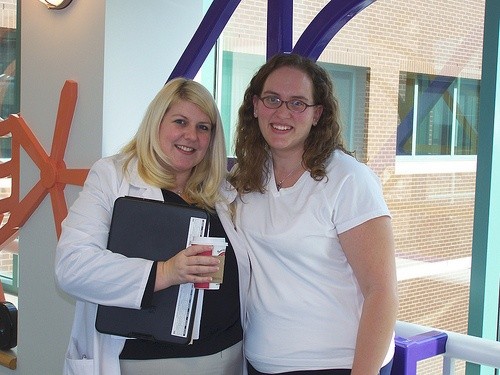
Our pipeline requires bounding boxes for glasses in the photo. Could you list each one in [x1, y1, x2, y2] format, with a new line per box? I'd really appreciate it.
[256, 95, 318, 113]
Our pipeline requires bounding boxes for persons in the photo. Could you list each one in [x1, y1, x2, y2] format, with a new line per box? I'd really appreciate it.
[211, 51, 399, 375]
[52, 77, 249, 375]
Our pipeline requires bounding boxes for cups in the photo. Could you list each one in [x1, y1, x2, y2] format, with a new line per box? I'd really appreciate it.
[190, 236, 228, 291]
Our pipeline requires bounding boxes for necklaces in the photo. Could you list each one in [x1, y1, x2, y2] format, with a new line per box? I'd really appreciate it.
[273, 159, 305, 190]
